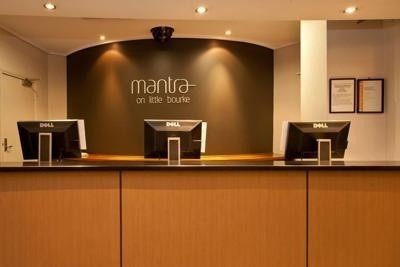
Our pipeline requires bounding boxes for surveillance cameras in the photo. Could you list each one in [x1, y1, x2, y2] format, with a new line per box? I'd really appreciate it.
[150, 26, 174, 43]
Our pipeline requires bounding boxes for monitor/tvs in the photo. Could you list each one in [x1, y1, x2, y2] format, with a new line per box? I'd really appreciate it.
[284, 121, 351, 165]
[144, 120, 203, 165]
[17, 121, 82, 167]
